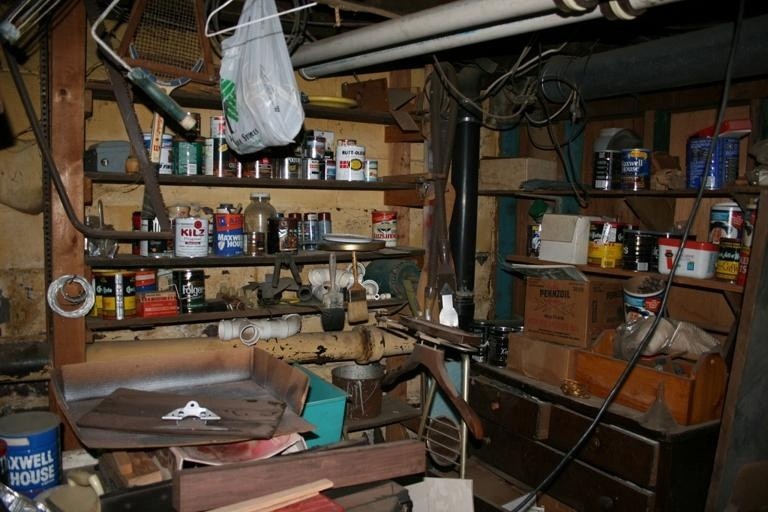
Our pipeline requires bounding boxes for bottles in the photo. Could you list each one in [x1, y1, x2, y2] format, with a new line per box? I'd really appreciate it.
[438, 294, 458, 327]
[131, 212, 141, 255]
[244, 193, 277, 253]
[288, 212, 303, 248]
[304, 213, 319, 250]
[318, 212, 332, 241]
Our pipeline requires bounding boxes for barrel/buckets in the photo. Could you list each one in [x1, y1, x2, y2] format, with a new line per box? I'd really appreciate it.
[622, 272, 669, 326]
[685, 137, 739, 189]
[332, 365, 385, 420]
[0, 411, 62, 499]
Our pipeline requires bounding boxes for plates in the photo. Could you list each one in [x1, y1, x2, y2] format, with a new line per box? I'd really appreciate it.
[307, 96, 358, 109]
[321, 234, 372, 243]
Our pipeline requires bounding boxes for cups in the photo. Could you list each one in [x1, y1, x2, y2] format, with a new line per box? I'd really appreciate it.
[167, 207, 192, 219]
[623, 275, 669, 321]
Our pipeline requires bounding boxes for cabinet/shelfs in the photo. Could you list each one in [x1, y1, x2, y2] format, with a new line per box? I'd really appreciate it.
[466, 352, 722, 511]
[508, 75, 768, 358]
[84, 72, 425, 334]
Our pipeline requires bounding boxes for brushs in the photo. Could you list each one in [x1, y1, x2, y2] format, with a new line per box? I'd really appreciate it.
[322, 252, 344, 332]
[348, 251, 368, 323]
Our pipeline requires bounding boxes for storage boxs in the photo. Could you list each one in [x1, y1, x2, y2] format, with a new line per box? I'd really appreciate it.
[300, 363, 352, 451]
[520, 267, 632, 351]
[506, 330, 578, 389]
[574, 326, 726, 425]
[537, 212, 619, 264]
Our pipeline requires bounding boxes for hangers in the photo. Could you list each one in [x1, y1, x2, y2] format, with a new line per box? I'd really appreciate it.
[203, 0, 319, 38]
[381, 342, 487, 442]
